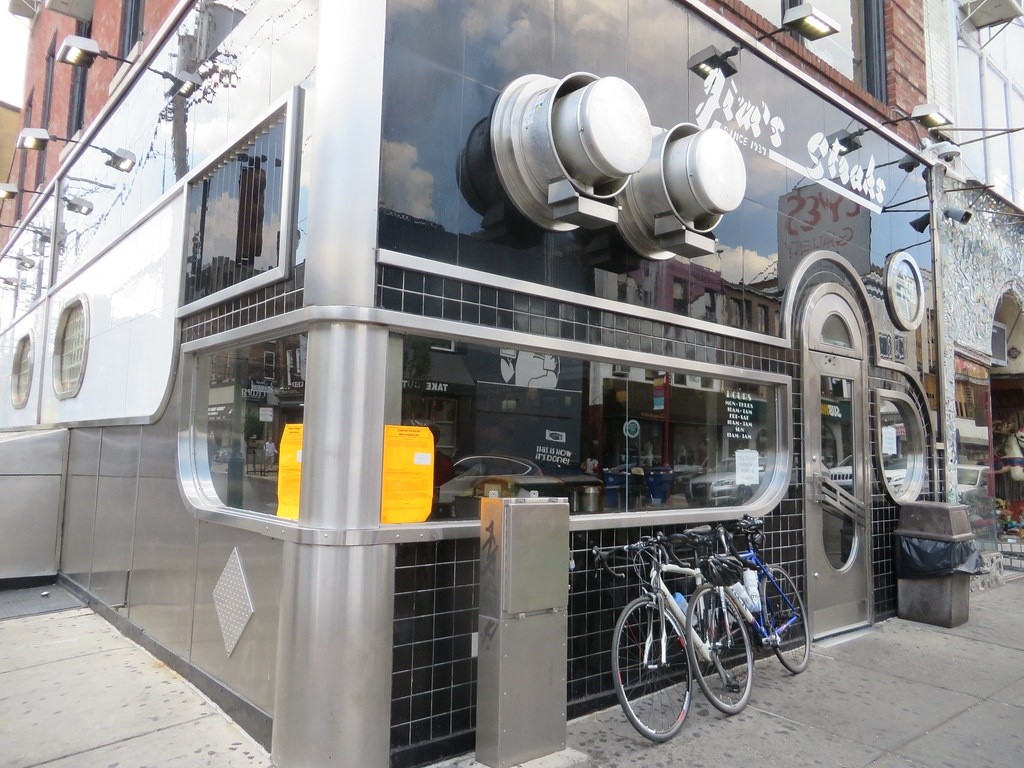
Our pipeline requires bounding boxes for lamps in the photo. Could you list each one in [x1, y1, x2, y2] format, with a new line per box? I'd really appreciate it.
[688, 45, 747, 80]
[25, 228, 67, 243]
[881, 103, 956, 128]
[15, 127, 78, 151]
[51, 193, 95, 215]
[826, 127, 871, 157]
[89, 143, 137, 173]
[0, 276, 19, 287]
[757, 3, 841, 42]
[4, 254, 35, 269]
[0, 182, 42, 199]
[54, 34, 134, 70]
[149, 68, 203, 99]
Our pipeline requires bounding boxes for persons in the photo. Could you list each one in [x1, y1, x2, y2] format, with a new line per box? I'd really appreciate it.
[263, 437, 278, 472]
[405, 419, 454, 519]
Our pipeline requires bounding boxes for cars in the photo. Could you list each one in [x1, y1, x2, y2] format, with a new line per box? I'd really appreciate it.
[432, 453, 604, 520]
[684, 456, 769, 507]
[609, 463, 703, 492]
[956, 464, 990, 503]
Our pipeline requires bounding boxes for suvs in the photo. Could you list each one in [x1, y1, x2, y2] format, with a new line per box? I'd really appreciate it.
[827, 454, 908, 495]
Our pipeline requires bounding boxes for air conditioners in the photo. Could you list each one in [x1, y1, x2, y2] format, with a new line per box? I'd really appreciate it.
[613, 364, 631, 374]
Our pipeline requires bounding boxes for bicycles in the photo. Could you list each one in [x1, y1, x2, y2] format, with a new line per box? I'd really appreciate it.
[591, 514, 811, 744]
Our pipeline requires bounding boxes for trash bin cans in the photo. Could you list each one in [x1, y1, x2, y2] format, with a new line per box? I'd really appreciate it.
[893, 500, 975, 628]
[604, 463, 673, 508]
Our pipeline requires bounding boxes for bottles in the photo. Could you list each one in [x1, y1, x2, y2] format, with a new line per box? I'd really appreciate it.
[568, 487, 601, 512]
[673, 592, 697, 627]
[728, 582, 755, 612]
[743, 568, 761, 612]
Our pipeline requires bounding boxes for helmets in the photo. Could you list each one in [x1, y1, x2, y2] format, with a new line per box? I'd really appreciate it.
[699, 556, 743, 586]
